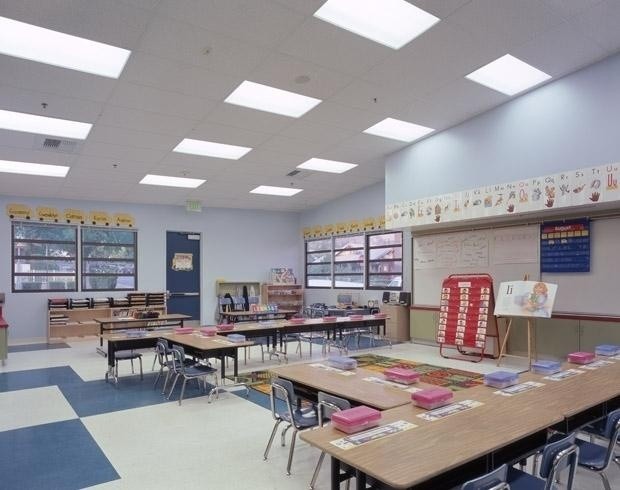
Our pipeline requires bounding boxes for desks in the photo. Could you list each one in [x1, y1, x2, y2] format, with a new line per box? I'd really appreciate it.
[298, 350, 619, 490]
[270, 353, 488, 412]
[0, 313, 10, 366]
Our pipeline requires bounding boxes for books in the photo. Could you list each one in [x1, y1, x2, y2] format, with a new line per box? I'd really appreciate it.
[46, 292, 167, 327]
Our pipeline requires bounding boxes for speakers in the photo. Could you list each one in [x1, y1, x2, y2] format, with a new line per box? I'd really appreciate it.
[382, 292, 411, 306]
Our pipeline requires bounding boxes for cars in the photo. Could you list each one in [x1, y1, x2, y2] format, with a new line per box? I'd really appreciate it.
[387, 276, 401, 288]
[13, 234, 135, 276]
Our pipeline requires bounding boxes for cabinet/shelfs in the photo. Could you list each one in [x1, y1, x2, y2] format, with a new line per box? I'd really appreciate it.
[47, 292, 167, 345]
[262, 283, 303, 314]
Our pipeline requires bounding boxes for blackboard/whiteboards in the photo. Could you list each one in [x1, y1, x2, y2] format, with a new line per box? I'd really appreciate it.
[410, 213, 620, 322]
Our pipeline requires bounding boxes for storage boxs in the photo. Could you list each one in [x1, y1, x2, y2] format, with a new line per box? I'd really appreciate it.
[567, 353, 596, 365]
[594, 344, 620, 356]
[327, 355, 358, 370]
[384, 367, 420, 385]
[330, 405, 382, 434]
[530, 360, 563, 376]
[411, 387, 453, 410]
[483, 370, 519, 389]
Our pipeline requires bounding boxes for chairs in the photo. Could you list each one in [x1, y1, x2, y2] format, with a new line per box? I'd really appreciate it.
[546, 410, 619, 490]
[92, 296, 390, 389]
[309, 391, 352, 490]
[165, 344, 218, 406]
[452, 463, 510, 490]
[506, 432, 580, 490]
[262, 377, 318, 476]
